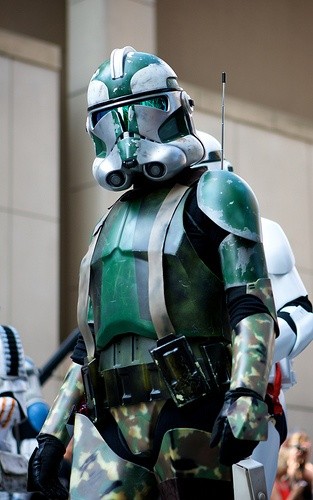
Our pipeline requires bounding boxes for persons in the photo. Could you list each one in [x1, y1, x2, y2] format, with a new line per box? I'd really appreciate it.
[192, 127, 312, 498]
[270, 430, 311, 500]
[0, 323, 73, 500]
[25, 46, 282, 500]
